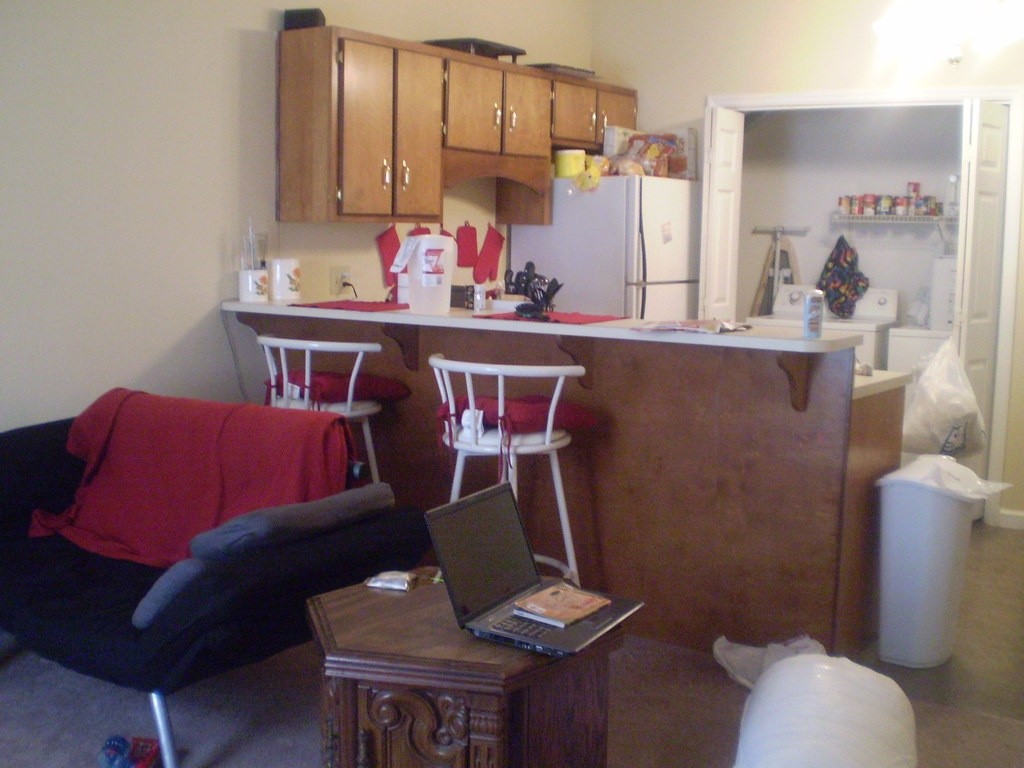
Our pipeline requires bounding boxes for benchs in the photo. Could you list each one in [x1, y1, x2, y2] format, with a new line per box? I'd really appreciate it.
[0, 387, 429, 768]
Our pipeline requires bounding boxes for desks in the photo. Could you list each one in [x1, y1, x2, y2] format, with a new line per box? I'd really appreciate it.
[308, 566, 626, 768]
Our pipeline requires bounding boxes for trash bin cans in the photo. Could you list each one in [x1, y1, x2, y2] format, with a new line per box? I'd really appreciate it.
[873, 454, 1015, 670]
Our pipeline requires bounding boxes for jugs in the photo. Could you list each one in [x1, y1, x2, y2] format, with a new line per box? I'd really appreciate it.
[406, 234, 459, 316]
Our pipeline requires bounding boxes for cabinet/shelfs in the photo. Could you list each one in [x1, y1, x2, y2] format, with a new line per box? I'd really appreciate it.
[277, 25, 638, 229]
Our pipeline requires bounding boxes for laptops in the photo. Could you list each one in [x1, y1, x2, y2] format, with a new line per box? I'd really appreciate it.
[425, 482, 647, 655]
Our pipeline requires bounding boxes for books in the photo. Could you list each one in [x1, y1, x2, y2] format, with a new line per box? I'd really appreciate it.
[514, 581, 610, 629]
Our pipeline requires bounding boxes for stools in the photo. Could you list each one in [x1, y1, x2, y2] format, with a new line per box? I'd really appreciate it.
[252, 334, 409, 483]
[430, 352, 602, 587]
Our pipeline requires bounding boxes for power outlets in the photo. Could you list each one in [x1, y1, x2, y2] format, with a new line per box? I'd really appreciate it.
[332, 255, 354, 295]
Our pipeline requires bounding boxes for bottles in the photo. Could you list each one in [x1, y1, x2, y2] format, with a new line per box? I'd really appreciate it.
[837, 183, 937, 218]
[464, 286, 489, 312]
[803, 288, 826, 336]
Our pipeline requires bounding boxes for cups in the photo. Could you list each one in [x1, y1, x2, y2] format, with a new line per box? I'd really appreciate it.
[553, 148, 585, 178]
[266, 258, 301, 306]
[397, 272, 410, 304]
[237, 269, 269, 307]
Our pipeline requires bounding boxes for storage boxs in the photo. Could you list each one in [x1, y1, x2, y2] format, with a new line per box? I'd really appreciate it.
[601, 124, 642, 154]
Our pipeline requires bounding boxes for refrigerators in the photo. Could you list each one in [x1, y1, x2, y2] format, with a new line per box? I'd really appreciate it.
[508, 177, 701, 322]
